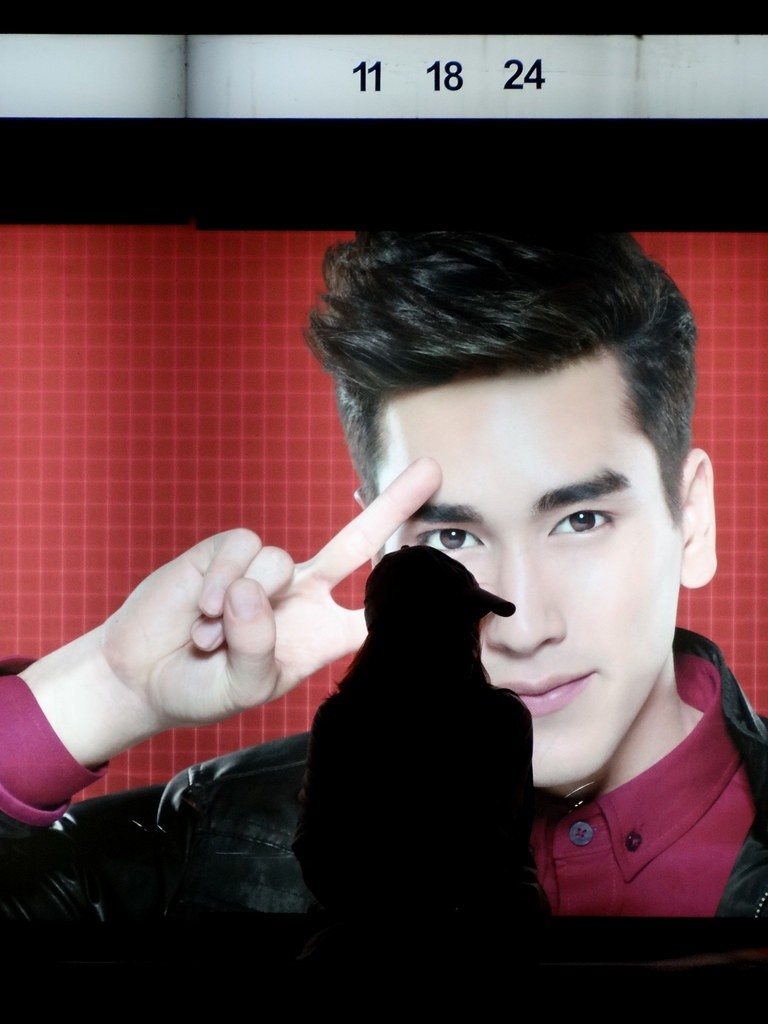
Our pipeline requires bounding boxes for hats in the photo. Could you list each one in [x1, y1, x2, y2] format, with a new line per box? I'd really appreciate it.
[364, 544, 516, 631]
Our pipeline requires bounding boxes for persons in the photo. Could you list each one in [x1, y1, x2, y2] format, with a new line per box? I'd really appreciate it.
[0, 229, 768, 924]
[295, 544, 553, 1024]
